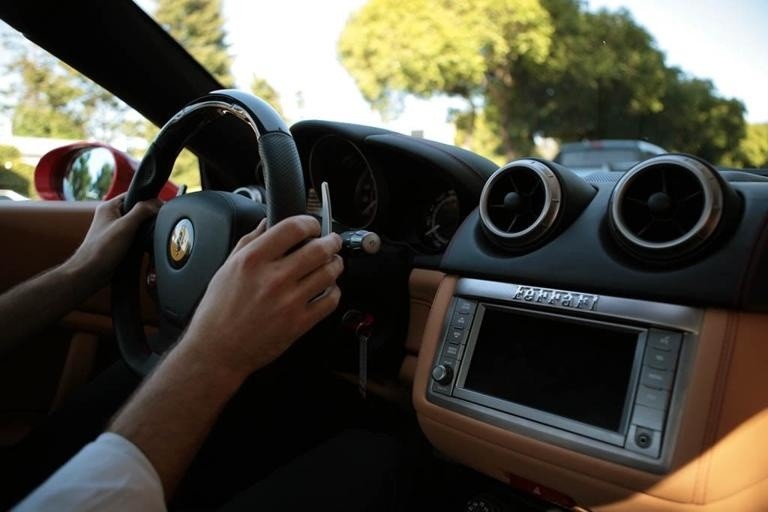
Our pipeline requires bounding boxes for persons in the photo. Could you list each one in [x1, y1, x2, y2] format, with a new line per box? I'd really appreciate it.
[2, 189, 346, 512]
